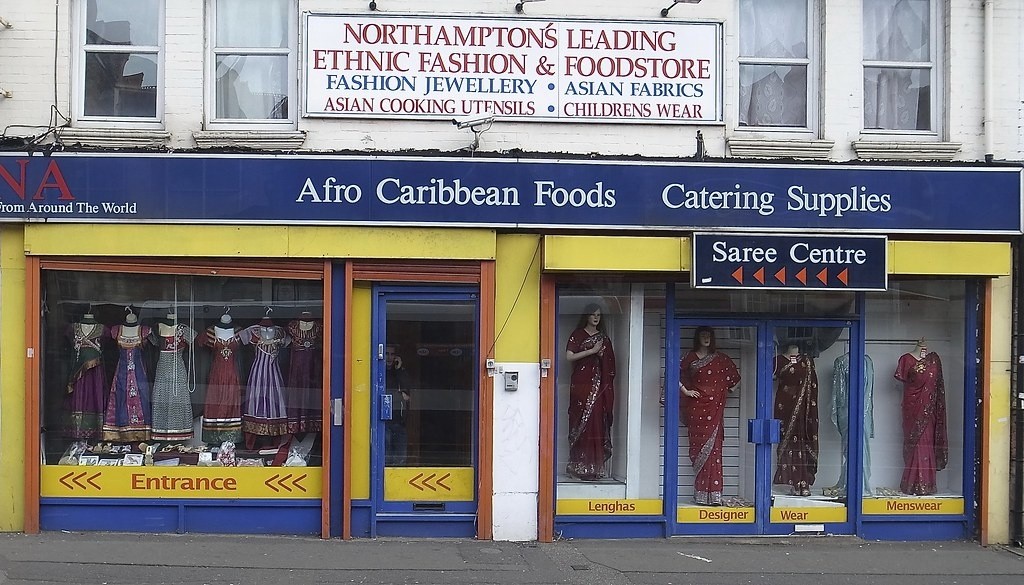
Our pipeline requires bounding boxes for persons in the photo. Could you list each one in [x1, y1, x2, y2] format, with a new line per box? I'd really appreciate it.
[199, 313, 245, 446]
[678, 325, 741, 507]
[773, 343, 821, 497]
[893, 341, 948, 495]
[386, 343, 411, 467]
[239, 314, 293, 438]
[286, 311, 323, 434]
[823, 340, 877, 496]
[66, 313, 105, 440]
[150, 313, 200, 441]
[565, 303, 617, 481]
[103, 313, 152, 444]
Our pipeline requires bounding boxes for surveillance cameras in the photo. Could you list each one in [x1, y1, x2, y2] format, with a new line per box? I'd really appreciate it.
[451, 112, 492, 129]
